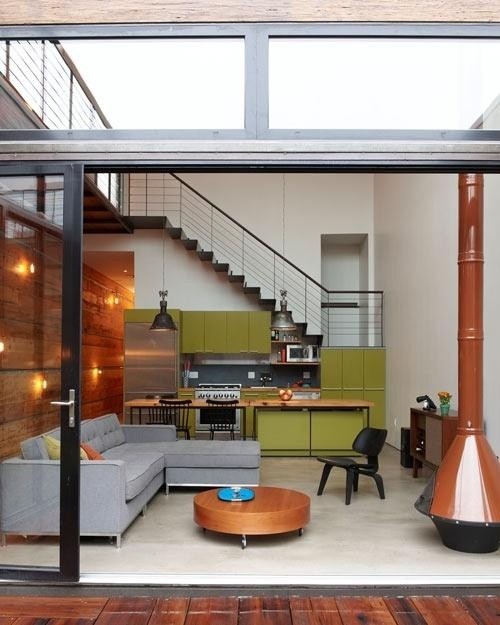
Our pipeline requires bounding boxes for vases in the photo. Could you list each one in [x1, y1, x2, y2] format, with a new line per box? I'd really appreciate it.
[439, 404, 450, 416]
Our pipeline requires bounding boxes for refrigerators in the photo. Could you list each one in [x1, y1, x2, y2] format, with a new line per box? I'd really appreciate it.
[123, 321, 180, 432]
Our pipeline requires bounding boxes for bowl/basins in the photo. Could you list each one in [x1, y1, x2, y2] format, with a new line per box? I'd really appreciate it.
[278, 393, 293, 401]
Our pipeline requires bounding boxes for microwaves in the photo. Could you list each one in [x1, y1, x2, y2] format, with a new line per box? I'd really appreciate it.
[287, 345, 321, 364]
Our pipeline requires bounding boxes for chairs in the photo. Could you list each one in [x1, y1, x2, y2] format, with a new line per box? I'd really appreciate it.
[206, 398, 239, 440]
[316, 427, 388, 504]
[145, 393, 192, 440]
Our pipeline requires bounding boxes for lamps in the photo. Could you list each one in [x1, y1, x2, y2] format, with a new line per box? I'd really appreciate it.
[269, 171, 298, 332]
[148, 174, 178, 331]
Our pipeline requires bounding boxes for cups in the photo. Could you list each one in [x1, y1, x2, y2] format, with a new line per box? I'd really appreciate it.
[233, 488, 241, 493]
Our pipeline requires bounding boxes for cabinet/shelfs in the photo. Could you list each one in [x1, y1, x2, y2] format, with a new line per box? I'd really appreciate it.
[256, 410, 311, 457]
[227, 311, 271, 354]
[271, 340, 320, 366]
[319, 347, 387, 430]
[241, 392, 280, 438]
[311, 410, 363, 456]
[180, 311, 227, 354]
[409, 406, 458, 478]
[179, 392, 195, 437]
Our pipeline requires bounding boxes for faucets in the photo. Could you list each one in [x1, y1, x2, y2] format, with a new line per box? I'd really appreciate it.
[259, 374, 273, 387]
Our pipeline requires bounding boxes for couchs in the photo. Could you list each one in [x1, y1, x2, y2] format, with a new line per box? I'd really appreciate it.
[1, 424, 261, 549]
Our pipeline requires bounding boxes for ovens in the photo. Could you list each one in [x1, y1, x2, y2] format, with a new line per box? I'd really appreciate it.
[196, 384, 241, 431]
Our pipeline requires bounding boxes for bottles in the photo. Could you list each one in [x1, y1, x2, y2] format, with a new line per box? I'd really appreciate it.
[271, 330, 281, 341]
[281, 350, 284, 362]
[283, 333, 298, 341]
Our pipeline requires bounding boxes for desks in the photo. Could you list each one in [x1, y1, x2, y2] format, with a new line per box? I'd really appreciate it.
[124, 398, 250, 441]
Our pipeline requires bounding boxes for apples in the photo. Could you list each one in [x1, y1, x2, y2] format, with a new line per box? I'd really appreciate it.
[279, 389, 293, 400]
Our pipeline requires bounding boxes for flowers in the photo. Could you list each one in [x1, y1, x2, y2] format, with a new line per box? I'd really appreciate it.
[438, 392, 452, 405]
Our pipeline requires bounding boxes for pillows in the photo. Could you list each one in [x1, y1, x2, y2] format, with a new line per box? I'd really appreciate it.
[39, 433, 105, 461]
